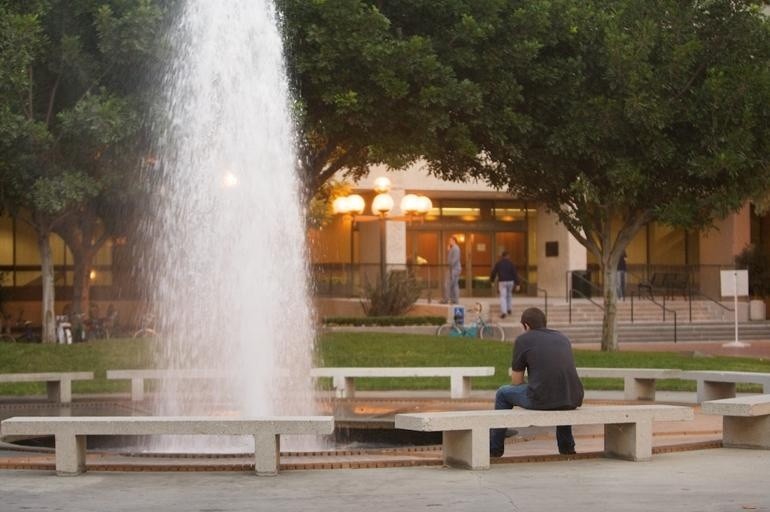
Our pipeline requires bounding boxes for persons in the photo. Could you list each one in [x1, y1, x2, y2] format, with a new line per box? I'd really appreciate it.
[616, 251, 628, 302]
[439, 237, 462, 304]
[491, 251, 515, 317]
[489, 306, 585, 457]
[63, 304, 120, 341]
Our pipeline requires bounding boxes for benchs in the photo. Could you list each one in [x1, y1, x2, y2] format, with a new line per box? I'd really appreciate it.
[0, 371, 94, 403]
[105, 370, 276, 402]
[509, 367, 681, 402]
[393, 404, 694, 470]
[701, 394, 770, 451]
[1, 415, 335, 478]
[682, 369, 770, 404]
[309, 366, 495, 399]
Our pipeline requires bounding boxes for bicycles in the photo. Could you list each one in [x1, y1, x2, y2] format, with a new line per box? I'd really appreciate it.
[1, 304, 163, 346]
[433, 302, 508, 343]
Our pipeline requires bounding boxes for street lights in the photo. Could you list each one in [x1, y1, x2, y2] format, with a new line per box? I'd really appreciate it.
[332, 177, 435, 294]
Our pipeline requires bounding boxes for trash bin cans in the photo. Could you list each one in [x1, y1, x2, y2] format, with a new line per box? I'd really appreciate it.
[572, 270, 592, 298]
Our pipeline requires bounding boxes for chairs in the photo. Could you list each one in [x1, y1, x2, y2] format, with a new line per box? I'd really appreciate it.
[638, 271, 690, 302]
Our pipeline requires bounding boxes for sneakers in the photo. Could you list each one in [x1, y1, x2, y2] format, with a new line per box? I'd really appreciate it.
[490, 451, 504, 457]
[560, 449, 577, 454]
[437, 298, 460, 304]
[500, 309, 512, 320]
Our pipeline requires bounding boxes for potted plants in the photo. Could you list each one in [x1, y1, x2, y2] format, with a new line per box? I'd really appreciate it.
[734, 241, 770, 321]
